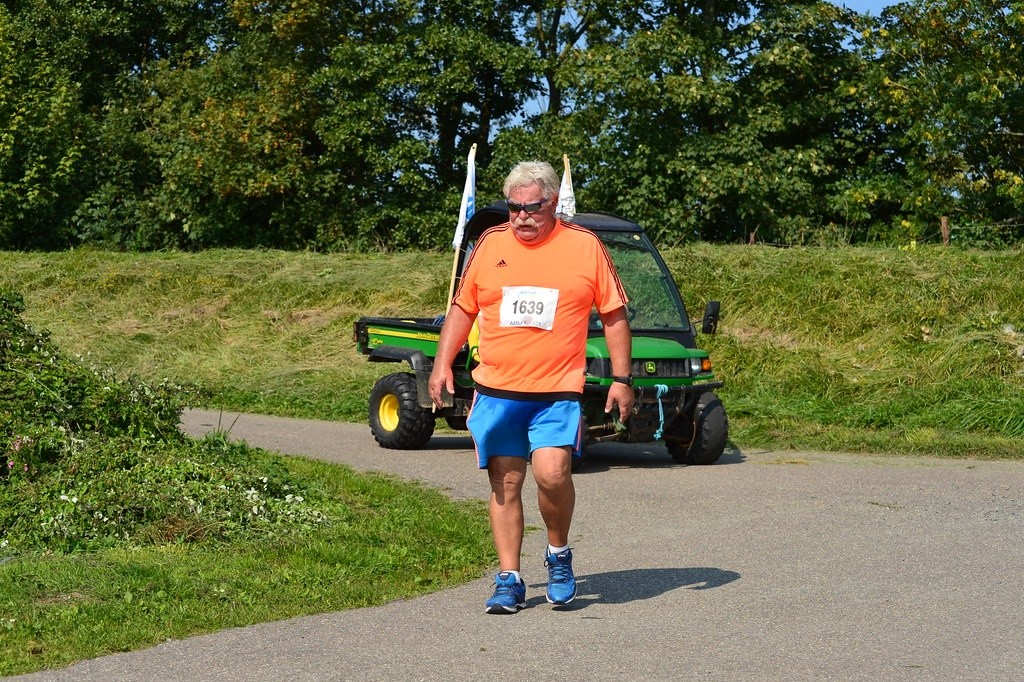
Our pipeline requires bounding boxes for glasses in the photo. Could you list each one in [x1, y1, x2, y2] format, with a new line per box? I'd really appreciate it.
[505, 197, 549, 213]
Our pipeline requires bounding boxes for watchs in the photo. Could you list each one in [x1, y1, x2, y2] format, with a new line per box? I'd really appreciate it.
[612, 374, 634, 387]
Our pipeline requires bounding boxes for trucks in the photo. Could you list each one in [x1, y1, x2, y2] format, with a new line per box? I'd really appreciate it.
[353, 202, 729, 463]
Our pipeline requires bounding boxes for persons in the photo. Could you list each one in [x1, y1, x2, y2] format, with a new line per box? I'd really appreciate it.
[428, 159, 635, 614]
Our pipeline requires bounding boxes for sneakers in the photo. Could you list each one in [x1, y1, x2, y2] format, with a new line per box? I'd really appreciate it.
[544, 545, 577, 606]
[485, 572, 527, 614]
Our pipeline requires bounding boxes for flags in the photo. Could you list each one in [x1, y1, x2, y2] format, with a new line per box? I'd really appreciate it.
[452, 143, 477, 251]
[555, 154, 576, 217]
